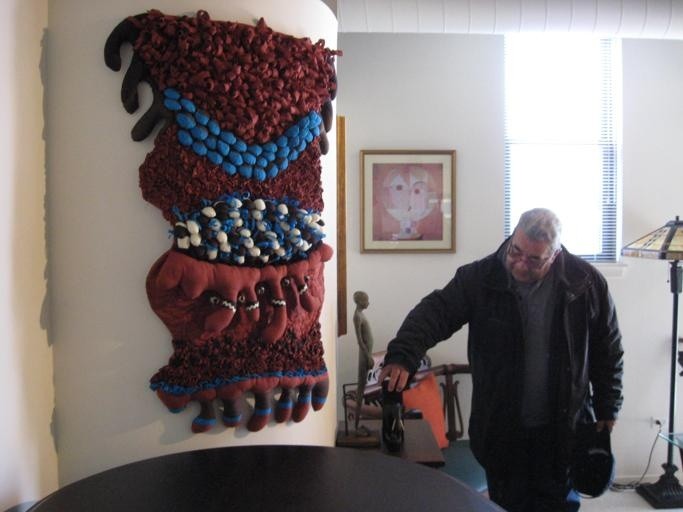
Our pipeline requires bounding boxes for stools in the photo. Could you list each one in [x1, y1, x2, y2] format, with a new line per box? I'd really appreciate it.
[434, 432, 493, 495]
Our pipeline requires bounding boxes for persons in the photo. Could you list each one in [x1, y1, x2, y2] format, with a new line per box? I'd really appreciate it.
[378, 208, 624, 512]
[352, 291, 374, 437]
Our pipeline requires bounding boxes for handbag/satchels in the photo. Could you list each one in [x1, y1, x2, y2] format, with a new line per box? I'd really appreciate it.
[568, 391, 615, 497]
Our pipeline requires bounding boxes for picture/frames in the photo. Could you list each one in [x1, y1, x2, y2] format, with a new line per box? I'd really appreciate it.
[357, 147, 458, 258]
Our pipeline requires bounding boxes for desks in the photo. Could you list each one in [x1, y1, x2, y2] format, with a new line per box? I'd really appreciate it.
[4, 434, 517, 511]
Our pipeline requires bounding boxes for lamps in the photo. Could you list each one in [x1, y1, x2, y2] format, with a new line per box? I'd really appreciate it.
[616, 203, 683, 510]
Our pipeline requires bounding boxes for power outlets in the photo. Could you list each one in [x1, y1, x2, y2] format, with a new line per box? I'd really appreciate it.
[650, 417, 666, 430]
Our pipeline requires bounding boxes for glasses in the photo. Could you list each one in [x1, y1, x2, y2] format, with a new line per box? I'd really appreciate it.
[508, 241, 552, 267]
